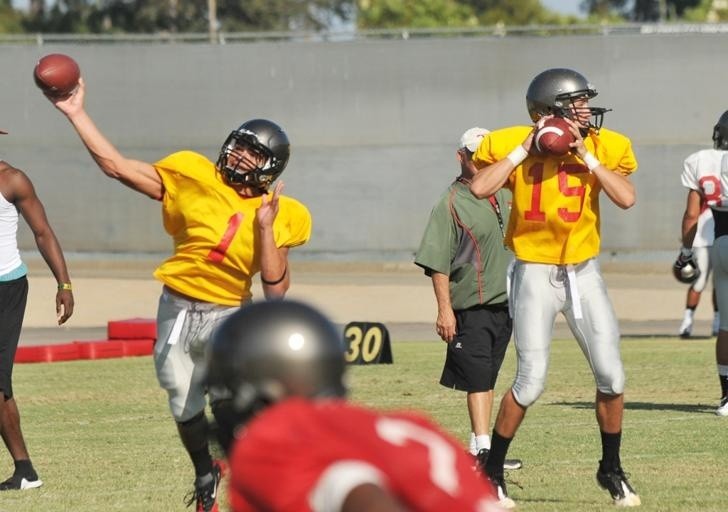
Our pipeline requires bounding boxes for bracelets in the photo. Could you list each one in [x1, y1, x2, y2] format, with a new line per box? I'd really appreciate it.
[56, 283, 72, 291]
[258, 266, 288, 286]
[582, 151, 601, 170]
[507, 144, 528, 168]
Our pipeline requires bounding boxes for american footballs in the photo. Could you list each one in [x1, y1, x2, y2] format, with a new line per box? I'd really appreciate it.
[535, 117, 575, 156]
[34, 54, 80, 95]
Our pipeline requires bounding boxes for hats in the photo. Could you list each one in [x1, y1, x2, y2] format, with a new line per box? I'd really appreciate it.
[459, 127, 491, 152]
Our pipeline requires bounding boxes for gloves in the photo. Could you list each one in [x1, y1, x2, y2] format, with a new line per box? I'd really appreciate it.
[672, 255, 701, 283]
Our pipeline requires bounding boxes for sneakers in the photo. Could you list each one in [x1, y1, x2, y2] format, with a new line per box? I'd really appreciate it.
[467, 449, 522, 470]
[679, 311, 720, 339]
[493, 479, 515, 508]
[184, 460, 226, 512]
[717, 401, 728, 416]
[0, 471, 43, 490]
[597, 460, 641, 507]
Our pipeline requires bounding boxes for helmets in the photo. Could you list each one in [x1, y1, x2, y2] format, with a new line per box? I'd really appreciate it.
[712, 110, 728, 151]
[215, 118, 291, 195]
[197, 299, 346, 452]
[525, 68, 612, 135]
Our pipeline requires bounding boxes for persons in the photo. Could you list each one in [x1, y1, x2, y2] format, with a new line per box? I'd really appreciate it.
[206, 298, 516, 512]
[0, 130, 74, 492]
[469, 69, 642, 509]
[43, 76, 312, 512]
[413, 127, 523, 471]
[671, 110, 728, 417]
[678, 200, 720, 337]
